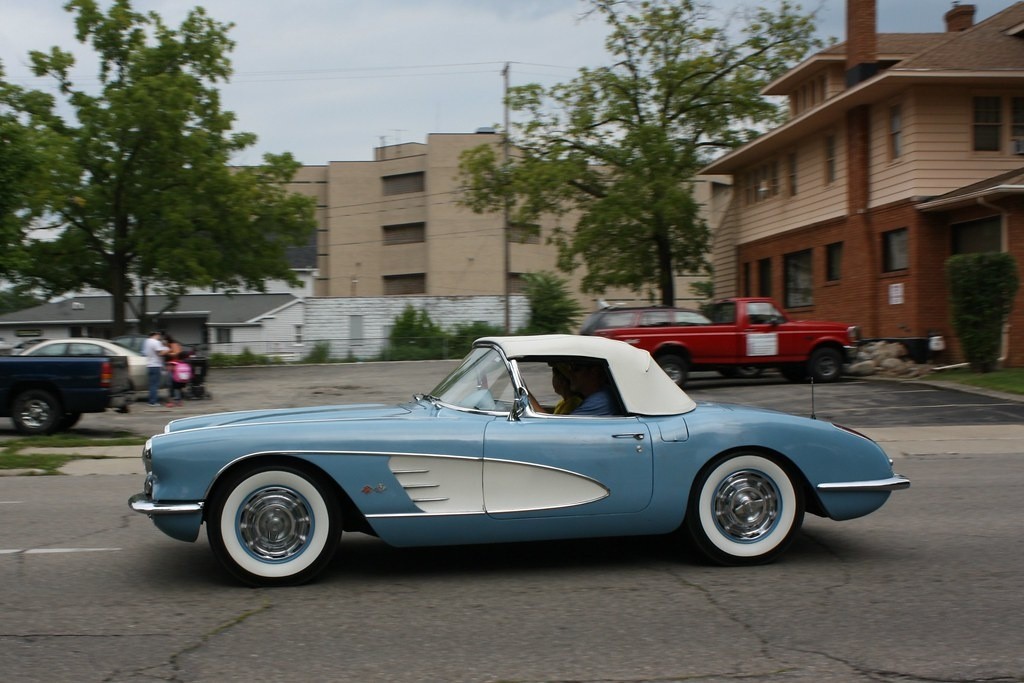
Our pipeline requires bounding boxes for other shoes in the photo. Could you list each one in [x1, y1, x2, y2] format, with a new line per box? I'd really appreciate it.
[148, 403, 161, 406]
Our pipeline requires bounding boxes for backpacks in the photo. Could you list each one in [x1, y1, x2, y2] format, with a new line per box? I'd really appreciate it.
[171, 362, 192, 383]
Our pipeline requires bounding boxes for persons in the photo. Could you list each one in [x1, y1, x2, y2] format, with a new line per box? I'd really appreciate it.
[142, 331, 168, 406]
[529, 357, 613, 415]
[161, 335, 186, 400]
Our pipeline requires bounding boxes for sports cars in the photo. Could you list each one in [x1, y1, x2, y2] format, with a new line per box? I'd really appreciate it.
[128, 334, 912, 588]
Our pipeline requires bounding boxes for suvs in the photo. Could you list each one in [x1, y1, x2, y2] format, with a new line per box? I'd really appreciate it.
[579, 306, 765, 380]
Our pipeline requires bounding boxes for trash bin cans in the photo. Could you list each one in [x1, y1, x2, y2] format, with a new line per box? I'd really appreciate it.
[183, 356, 208, 399]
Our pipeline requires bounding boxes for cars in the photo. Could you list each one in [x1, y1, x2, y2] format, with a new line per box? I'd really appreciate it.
[2, 337, 167, 406]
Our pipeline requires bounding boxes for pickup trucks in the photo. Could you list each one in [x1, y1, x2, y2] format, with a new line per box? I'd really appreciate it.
[591, 298, 862, 392]
[0, 353, 130, 435]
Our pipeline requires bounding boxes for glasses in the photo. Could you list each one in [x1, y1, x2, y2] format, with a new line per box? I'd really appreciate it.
[569, 364, 599, 373]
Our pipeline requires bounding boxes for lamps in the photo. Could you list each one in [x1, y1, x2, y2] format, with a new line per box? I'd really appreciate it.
[756, 179, 778, 192]
